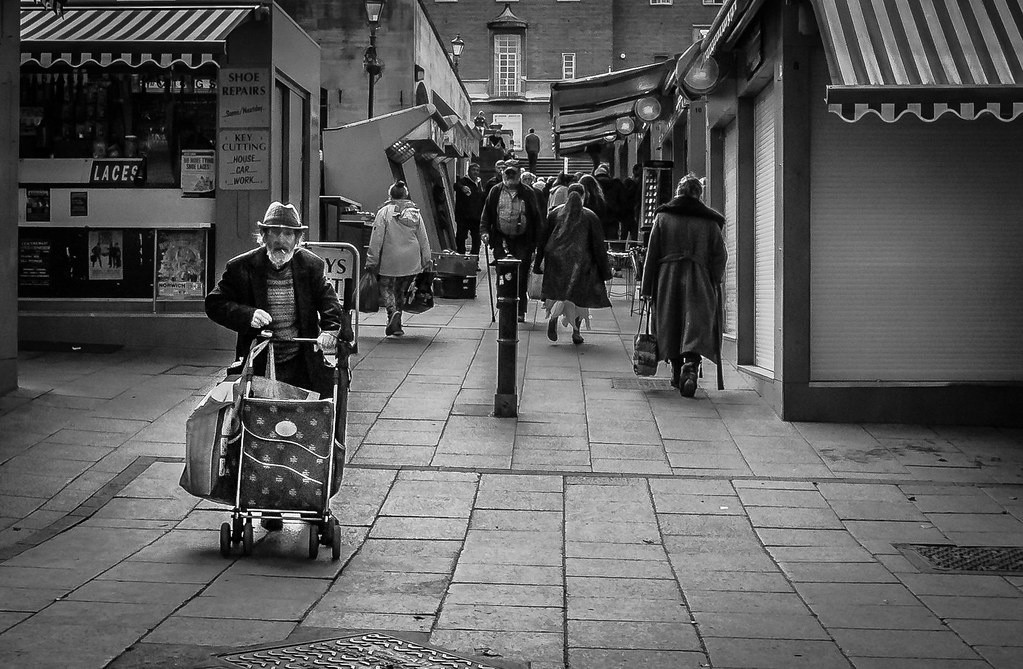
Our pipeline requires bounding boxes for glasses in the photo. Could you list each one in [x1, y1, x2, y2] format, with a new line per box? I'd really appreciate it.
[523, 178, 530, 180]
[506, 172, 517, 175]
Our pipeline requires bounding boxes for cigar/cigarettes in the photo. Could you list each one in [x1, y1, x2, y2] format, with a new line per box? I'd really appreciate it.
[280, 248, 286, 255]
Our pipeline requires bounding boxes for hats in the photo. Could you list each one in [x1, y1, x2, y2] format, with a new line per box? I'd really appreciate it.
[504, 159, 520, 173]
[256, 201, 309, 230]
[495, 160, 506, 167]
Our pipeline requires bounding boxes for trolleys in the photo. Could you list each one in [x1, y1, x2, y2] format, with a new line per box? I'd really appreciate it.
[220, 330, 341, 561]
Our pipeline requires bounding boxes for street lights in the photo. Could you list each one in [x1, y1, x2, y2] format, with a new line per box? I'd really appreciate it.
[449, 32, 466, 68]
[363, 0, 386, 116]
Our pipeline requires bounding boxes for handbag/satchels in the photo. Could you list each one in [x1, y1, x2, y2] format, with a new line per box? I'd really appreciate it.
[186, 346, 242, 495]
[351, 269, 379, 312]
[252, 341, 321, 400]
[404, 266, 436, 314]
[633, 299, 658, 376]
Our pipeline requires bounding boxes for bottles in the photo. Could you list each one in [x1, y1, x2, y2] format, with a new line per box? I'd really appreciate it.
[124, 135, 137, 158]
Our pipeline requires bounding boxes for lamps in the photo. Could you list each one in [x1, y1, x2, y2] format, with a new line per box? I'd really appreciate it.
[616, 116, 636, 136]
[633, 96, 663, 124]
[681, 55, 722, 96]
[451, 32, 466, 72]
[414, 65, 424, 82]
[603, 130, 618, 142]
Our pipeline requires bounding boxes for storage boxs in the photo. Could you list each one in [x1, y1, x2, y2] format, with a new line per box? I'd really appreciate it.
[431, 252, 479, 299]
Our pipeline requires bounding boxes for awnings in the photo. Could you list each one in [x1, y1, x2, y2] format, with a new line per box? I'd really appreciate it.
[20, 5, 266, 68]
[814, 0, 1023, 122]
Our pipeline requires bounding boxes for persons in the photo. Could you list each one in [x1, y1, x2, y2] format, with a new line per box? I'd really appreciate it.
[525, 129, 541, 174]
[203, 202, 343, 531]
[456, 164, 482, 272]
[480, 159, 622, 274]
[365, 181, 432, 336]
[487, 129, 505, 150]
[620, 164, 643, 250]
[474, 112, 488, 136]
[537, 184, 613, 343]
[479, 159, 541, 322]
[639, 171, 725, 397]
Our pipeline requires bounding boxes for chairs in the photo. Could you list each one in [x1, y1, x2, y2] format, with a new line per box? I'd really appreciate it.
[630, 248, 648, 315]
[606, 251, 631, 300]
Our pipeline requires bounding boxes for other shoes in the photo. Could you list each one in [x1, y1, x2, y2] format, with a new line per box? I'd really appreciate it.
[385, 309, 403, 336]
[548, 318, 558, 341]
[572, 333, 584, 343]
[669, 362, 685, 387]
[518, 311, 524, 322]
[614, 270, 622, 277]
[533, 266, 543, 274]
[679, 362, 698, 397]
[261, 513, 283, 531]
[301, 508, 339, 526]
